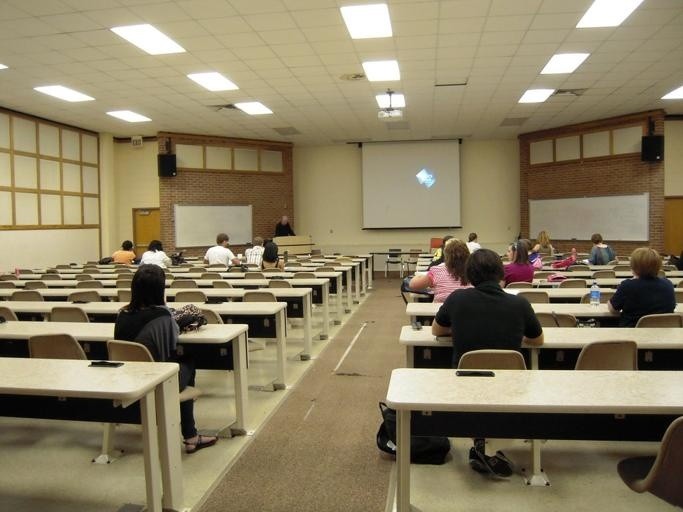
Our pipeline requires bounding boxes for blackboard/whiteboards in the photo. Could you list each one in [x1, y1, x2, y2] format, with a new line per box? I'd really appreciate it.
[529, 195, 651, 242]
[174, 204, 252, 248]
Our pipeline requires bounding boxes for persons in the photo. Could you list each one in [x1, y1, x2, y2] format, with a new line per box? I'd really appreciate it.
[589, 234, 615, 265]
[524, 238, 542, 262]
[274, 215, 296, 237]
[435, 245, 443, 256]
[139, 240, 172, 268]
[203, 233, 240, 267]
[427, 236, 454, 271]
[112, 240, 137, 265]
[531, 231, 553, 256]
[465, 232, 481, 254]
[431, 249, 544, 478]
[113, 264, 219, 454]
[262, 242, 284, 272]
[499, 240, 534, 288]
[245, 237, 266, 269]
[607, 247, 676, 328]
[410, 241, 475, 304]
[438, 238, 461, 266]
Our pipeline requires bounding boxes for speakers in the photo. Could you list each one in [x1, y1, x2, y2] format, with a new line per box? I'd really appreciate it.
[642, 135, 664, 160]
[156, 153, 176, 179]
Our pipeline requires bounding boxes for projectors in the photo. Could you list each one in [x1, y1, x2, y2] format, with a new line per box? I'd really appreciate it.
[379, 91, 404, 121]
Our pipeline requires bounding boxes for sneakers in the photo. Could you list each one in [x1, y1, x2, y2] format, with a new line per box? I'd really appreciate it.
[469, 448, 490, 474]
[474, 441, 513, 478]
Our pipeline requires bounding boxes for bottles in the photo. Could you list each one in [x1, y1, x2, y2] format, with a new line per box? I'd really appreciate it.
[571, 248, 577, 262]
[590, 282, 600, 312]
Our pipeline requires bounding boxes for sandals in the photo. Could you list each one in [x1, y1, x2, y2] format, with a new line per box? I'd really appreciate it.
[183, 435, 218, 454]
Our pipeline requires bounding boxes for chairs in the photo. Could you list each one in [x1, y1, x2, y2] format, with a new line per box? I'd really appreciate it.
[569, 265, 591, 272]
[673, 292, 683, 304]
[311, 255, 324, 258]
[0, 282, 15, 289]
[40, 274, 61, 280]
[15, 269, 33, 274]
[199, 308, 225, 325]
[201, 272, 222, 280]
[75, 275, 94, 281]
[117, 274, 134, 280]
[534, 312, 578, 328]
[262, 267, 282, 272]
[227, 266, 245, 273]
[27, 334, 88, 361]
[241, 264, 259, 268]
[24, 282, 48, 290]
[209, 264, 226, 268]
[612, 265, 631, 272]
[0, 307, 18, 322]
[457, 349, 527, 371]
[188, 267, 207, 273]
[45, 269, 59, 274]
[385, 248, 402, 279]
[10, 291, 44, 302]
[212, 281, 232, 289]
[82, 268, 100, 274]
[293, 272, 316, 279]
[505, 282, 533, 289]
[82, 265, 97, 269]
[515, 291, 549, 304]
[163, 273, 175, 280]
[316, 267, 335, 272]
[114, 270, 132, 274]
[242, 292, 278, 302]
[55, 264, 72, 269]
[404, 249, 423, 276]
[324, 262, 341, 266]
[335, 256, 352, 263]
[101, 339, 202, 464]
[268, 281, 292, 288]
[635, 313, 683, 329]
[0, 275, 17, 281]
[574, 341, 638, 371]
[114, 264, 131, 269]
[660, 264, 678, 272]
[76, 281, 103, 288]
[676, 280, 683, 288]
[617, 416, 683, 509]
[287, 254, 297, 259]
[170, 280, 198, 288]
[244, 272, 265, 280]
[162, 267, 170, 272]
[580, 291, 616, 304]
[116, 281, 132, 289]
[533, 272, 557, 279]
[295, 259, 312, 263]
[87, 262, 97, 265]
[50, 307, 90, 323]
[117, 290, 132, 302]
[541, 263, 552, 266]
[180, 264, 194, 268]
[174, 291, 208, 303]
[285, 262, 301, 267]
[67, 291, 102, 303]
[591, 271, 615, 279]
[558, 280, 587, 289]
[540, 256, 557, 262]
[615, 256, 630, 262]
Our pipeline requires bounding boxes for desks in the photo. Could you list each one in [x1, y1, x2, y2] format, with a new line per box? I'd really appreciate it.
[183, 258, 367, 297]
[0, 301, 288, 392]
[0, 357, 183, 512]
[413, 254, 683, 286]
[0, 320, 249, 439]
[184, 262, 360, 304]
[385, 367, 683, 512]
[30, 268, 343, 325]
[406, 302, 683, 325]
[0, 274, 330, 341]
[0, 287, 314, 361]
[409, 288, 683, 302]
[67, 265, 353, 313]
[180, 254, 373, 290]
[399, 325, 683, 487]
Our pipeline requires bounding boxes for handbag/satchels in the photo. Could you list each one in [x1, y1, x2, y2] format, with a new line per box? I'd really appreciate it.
[400, 274, 434, 305]
[551, 255, 576, 268]
[99, 256, 112, 265]
[226, 264, 248, 272]
[169, 303, 207, 333]
[170, 249, 187, 266]
[547, 274, 567, 282]
